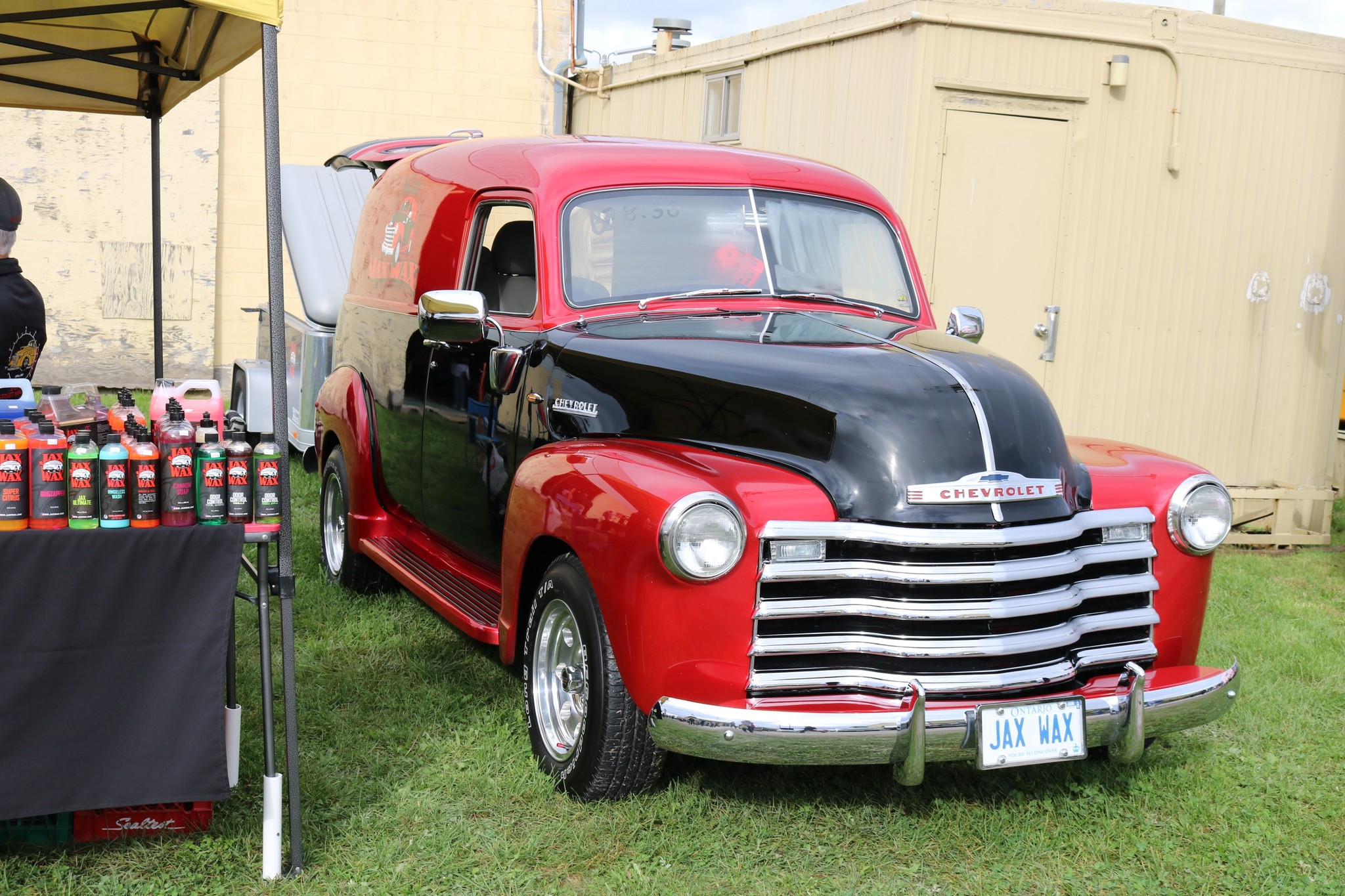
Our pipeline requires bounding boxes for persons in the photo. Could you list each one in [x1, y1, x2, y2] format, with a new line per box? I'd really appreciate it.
[0, 178, 46, 399]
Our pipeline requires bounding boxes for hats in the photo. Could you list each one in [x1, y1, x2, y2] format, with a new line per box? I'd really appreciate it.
[0, 177, 22, 231]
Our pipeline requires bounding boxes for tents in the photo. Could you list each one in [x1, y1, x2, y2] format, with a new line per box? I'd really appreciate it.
[0, 0, 303, 448]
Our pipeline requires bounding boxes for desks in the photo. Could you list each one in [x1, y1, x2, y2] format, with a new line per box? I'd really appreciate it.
[0, 515, 281, 883]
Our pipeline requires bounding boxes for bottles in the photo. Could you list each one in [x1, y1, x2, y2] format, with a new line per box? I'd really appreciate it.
[252, 433, 282, 524]
[13, 408, 38, 429]
[108, 387, 146, 434]
[196, 433, 226, 526]
[68, 435, 99, 529]
[203, 411, 211, 420]
[220, 430, 236, 449]
[0, 419, 28, 531]
[225, 432, 253, 524]
[21, 414, 45, 437]
[78, 429, 99, 473]
[76, 433, 99, 486]
[155, 397, 197, 526]
[120, 413, 160, 528]
[17, 411, 42, 430]
[28, 422, 69, 529]
[26, 419, 64, 435]
[195, 419, 219, 459]
[99, 434, 130, 528]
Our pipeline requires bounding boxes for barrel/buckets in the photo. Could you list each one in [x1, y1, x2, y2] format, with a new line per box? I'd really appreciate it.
[149, 378, 224, 446]
[0, 378, 36, 422]
[36, 382, 109, 453]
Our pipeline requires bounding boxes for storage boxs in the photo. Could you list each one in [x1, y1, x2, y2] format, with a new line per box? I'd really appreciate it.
[0, 802, 213, 854]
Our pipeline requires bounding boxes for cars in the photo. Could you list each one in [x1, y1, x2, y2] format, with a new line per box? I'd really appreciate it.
[301, 129, 1243, 804]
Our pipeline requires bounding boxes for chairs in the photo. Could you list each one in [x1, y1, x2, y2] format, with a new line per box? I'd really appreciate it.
[474, 221, 610, 313]
